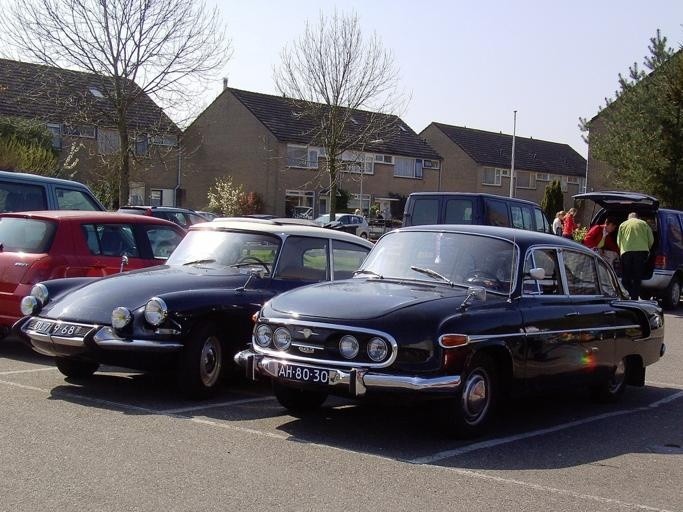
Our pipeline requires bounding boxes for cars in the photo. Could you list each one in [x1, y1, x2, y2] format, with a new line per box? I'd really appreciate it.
[0, 211, 190, 355]
[103, 205, 401, 246]
[232, 222, 666, 441]
[15, 215, 375, 398]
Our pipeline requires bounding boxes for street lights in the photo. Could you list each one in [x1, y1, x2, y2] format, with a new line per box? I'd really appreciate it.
[358, 140, 382, 215]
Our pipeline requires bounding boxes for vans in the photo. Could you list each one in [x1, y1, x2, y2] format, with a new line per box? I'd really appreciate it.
[568, 190, 682, 309]
[402, 192, 562, 281]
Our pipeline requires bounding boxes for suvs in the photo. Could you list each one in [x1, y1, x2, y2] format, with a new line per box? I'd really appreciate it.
[0, 170, 134, 259]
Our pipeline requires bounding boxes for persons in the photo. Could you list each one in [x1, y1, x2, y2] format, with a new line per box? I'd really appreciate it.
[615, 211, 652, 301]
[581, 216, 616, 252]
[562, 208, 580, 239]
[376, 210, 384, 223]
[351, 209, 365, 222]
[552, 210, 565, 238]
